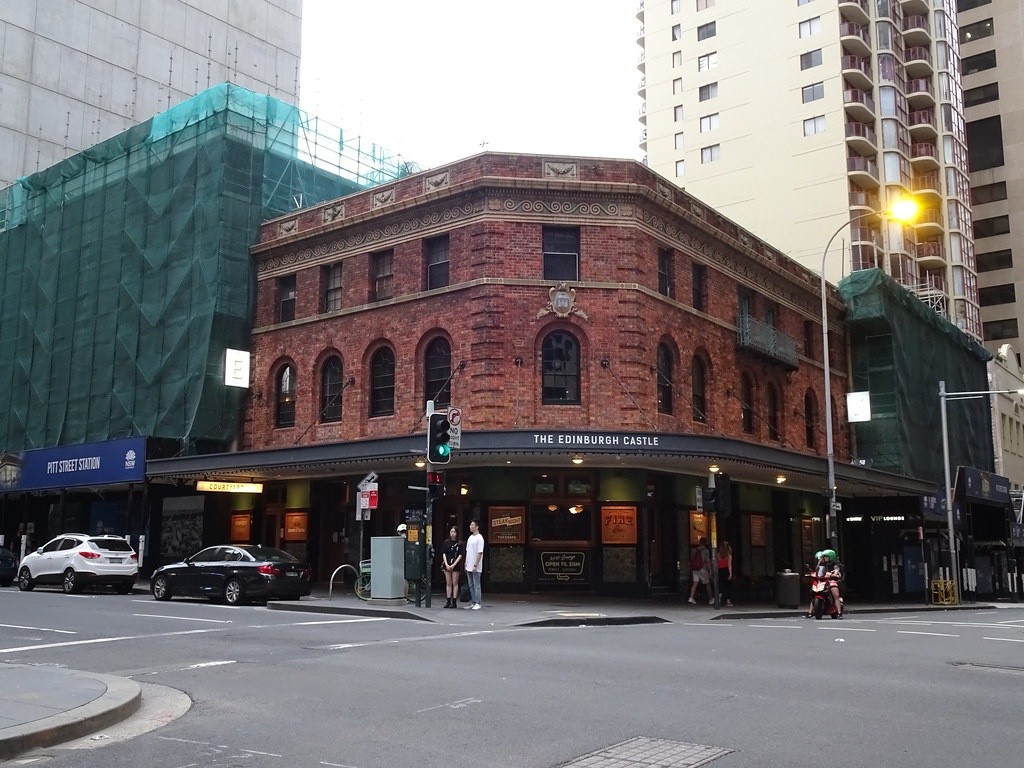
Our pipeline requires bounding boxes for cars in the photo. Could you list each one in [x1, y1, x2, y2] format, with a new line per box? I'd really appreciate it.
[151, 544, 315, 603]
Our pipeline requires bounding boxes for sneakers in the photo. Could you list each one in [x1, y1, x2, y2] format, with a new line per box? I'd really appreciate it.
[472, 603, 481, 610]
[463, 604, 474, 609]
[708, 598, 715, 604]
[689, 597, 698, 604]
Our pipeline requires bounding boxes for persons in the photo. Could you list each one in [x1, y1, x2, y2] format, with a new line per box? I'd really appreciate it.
[396, 523, 408, 538]
[806, 550, 844, 619]
[715, 539, 735, 606]
[801, 550, 842, 615]
[685, 537, 716, 605]
[439, 525, 465, 608]
[462, 519, 485, 610]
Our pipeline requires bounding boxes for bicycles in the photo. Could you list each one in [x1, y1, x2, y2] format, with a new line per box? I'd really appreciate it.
[355, 559, 427, 604]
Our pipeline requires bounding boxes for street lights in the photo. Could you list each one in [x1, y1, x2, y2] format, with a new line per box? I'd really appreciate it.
[822, 196, 924, 562]
[940, 381, 1024, 605]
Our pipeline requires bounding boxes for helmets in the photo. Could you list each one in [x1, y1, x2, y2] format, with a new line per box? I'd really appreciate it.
[815, 551, 824, 560]
[822, 549, 837, 562]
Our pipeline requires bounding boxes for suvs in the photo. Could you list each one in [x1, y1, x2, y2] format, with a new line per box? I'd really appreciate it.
[18, 532, 139, 594]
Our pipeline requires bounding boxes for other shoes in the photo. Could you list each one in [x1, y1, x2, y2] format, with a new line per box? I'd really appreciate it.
[443, 603, 451, 607]
[837, 614, 843, 619]
[449, 604, 456, 608]
[805, 614, 813, 618]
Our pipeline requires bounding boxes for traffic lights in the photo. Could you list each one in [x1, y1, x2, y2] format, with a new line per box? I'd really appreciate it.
[428, 413, 451, 464]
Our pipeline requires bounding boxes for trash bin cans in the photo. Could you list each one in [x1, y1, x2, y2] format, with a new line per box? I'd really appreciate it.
[777, 570, 802, 609]
